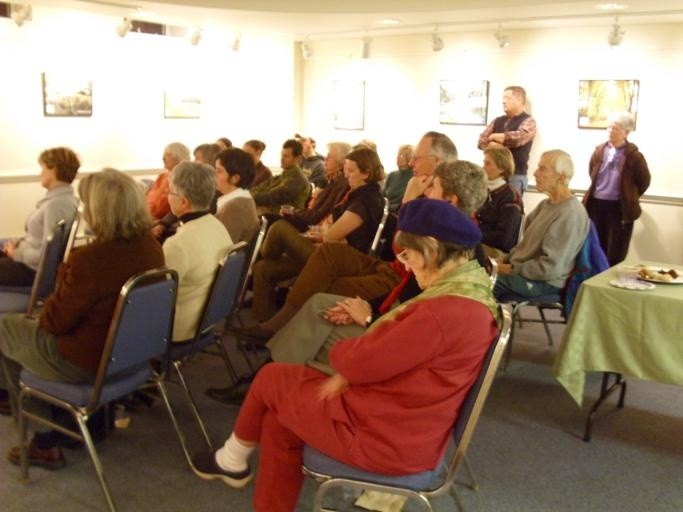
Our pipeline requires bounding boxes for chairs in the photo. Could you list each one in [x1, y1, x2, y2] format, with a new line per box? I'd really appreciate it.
[225, 218, 270, 371]
[302, 304, 512, 512]
[164, 241, 251, 449]
[0, 221, 65, 318]
[368, 197, 389, 256]
[510, 295, 563, 344]
[305, 183, 315, 208]
[14, 269, 193, 512]
[486, 257, 498, 290]
[0, 204, 83, 318]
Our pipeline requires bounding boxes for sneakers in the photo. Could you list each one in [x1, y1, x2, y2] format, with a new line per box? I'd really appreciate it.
[190, 452, 253, 489]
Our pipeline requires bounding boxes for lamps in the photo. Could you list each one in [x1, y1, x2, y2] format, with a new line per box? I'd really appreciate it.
[362, 38, 372, 58]
[495, 29, 510, 50]
[12, 4, 32, 27]
[232, 35, 241, 52]
[191, 28, 202, 44]
[431, 34, 444, 50]
[116, 19, 132, 37]
[609, 25, 625, 46]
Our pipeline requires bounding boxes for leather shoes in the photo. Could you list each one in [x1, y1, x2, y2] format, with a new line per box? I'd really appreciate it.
[0, 397, 11, 416]
[227, 323, 274, 342]
[205, 376, 251, 406]
[8, 439, 67, 470]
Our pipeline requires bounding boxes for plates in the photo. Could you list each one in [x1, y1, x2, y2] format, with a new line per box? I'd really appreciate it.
[635, 266, 682, 285]
[609, 278, 656, 292]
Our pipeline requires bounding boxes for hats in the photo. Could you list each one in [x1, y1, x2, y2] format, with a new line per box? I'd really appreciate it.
[398, 199, 483, 245]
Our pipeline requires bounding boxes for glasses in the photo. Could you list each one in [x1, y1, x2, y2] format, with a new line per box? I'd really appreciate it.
[412, 154, 435, 162]
[398, 250, 434, 265]
[163, 187, 180, 199]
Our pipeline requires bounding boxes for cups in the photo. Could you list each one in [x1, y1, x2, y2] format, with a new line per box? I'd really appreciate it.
[281, 205, 294, 215]
[616, 265, 639, 284]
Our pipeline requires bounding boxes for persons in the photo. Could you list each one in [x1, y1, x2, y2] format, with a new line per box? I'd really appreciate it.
[189, 195, 496, 512]
[492, 152, 591, 305]
[582, 109, 651, 270]
[7, 169, 165, 472]
[476, 86, 537, 196]
[0, 132, 521, 410]
